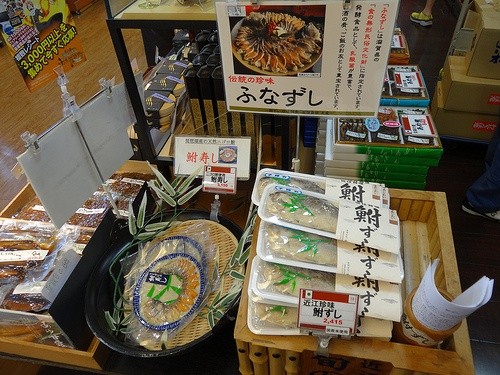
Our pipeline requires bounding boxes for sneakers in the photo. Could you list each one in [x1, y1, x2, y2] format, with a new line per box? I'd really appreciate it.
[410, 11, 433, 26]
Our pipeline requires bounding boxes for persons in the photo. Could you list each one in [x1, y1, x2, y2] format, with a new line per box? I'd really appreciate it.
[409, 0, 436, 27]
[462, 133, 500, 223]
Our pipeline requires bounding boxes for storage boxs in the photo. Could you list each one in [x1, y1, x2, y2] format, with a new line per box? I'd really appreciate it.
[183, 28, 444, 191]
[0, 161, 157, 370]
[431, 0, 500, 141]
[234, 188, 477, 375]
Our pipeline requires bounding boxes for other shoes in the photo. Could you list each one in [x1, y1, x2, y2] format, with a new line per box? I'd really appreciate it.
[462, 196, 500, 222]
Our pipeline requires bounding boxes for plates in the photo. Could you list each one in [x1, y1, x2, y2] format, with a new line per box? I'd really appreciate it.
[231, 18, 323, 76]
[247, 168, 403, 339]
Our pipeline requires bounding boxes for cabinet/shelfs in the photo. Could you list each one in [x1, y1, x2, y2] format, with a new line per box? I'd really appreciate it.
[104, 0, 290, 187]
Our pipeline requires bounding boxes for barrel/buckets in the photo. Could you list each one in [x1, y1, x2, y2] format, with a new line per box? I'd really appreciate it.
[83, 206, 249, 374]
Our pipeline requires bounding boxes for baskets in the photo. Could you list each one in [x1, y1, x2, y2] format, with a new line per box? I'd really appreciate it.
[122, 219, 245, 351]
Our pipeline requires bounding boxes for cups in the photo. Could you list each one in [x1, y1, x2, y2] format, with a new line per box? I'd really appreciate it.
[393, 286, 462, 348]
